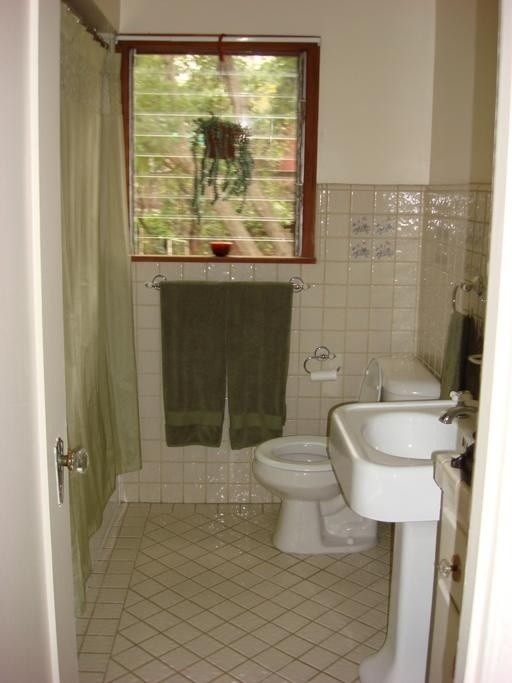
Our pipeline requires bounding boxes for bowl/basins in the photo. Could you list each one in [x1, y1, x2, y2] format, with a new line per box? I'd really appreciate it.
[210, 242, 233, 255]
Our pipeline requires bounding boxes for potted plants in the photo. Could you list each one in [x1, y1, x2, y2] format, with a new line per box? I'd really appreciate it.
[188, 110, 253, 230]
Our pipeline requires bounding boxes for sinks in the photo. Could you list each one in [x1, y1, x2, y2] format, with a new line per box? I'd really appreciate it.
[325, 399, 477, 522]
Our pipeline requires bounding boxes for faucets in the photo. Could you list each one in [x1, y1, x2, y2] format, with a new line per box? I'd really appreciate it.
[437, 404, 478, 424]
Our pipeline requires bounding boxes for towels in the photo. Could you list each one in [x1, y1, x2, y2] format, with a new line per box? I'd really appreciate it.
[157, 279, 287, 445]
[440, 308, 468, 404]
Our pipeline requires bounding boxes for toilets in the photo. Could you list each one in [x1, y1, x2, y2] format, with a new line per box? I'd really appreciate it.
[252, 356, 442, 556]
